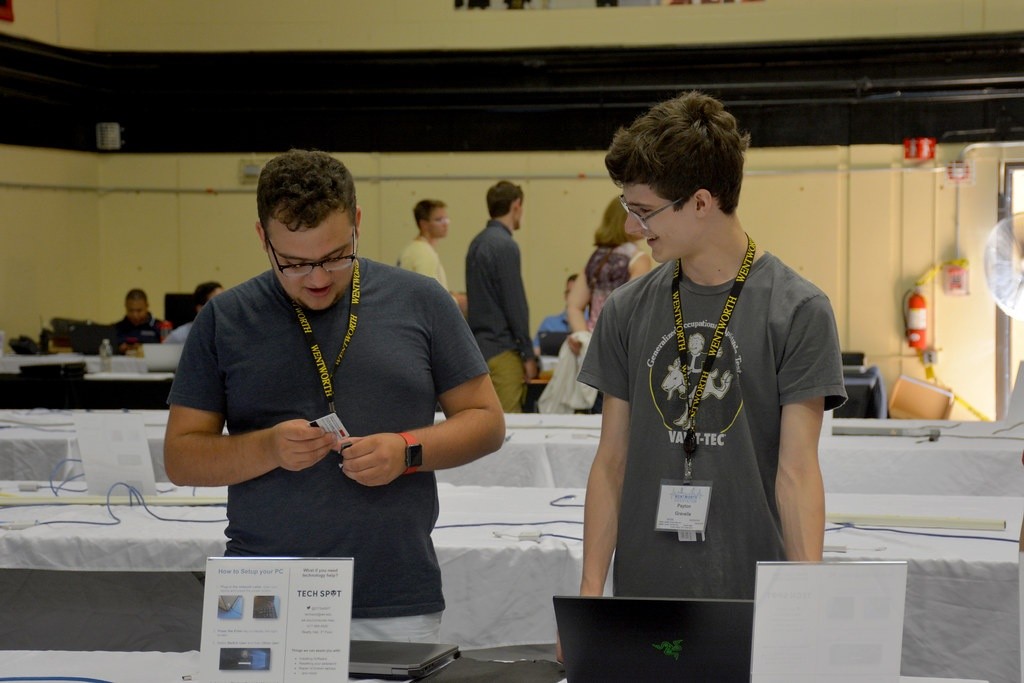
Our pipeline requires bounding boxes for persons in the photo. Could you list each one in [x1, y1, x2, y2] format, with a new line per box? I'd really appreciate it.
[109, 180, 652, 412]
[556, 91, 847, 664]
[163, 149, 506, 644]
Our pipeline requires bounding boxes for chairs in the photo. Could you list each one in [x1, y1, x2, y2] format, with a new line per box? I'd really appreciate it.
[82, 373, 174, 411]
[22, 363, 86, 410]
[886, 374, 956, 420]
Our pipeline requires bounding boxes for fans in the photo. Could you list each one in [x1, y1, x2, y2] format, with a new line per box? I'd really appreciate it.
[984, 213, 1024, 321]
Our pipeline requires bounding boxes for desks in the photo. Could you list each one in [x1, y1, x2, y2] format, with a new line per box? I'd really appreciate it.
[0, 410, 1024, 497]
[1, 650, 990, 683]
[0, 352, 147, 410]
[529, 367, 887, 420]
[0, 480, 1024, 683]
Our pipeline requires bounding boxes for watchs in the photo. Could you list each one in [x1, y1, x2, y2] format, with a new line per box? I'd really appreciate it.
[395, 432, 422, 475]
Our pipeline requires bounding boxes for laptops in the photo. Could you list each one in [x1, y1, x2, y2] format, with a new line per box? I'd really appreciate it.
[349, 639, 459, 679]
[554, 595, 755, 683]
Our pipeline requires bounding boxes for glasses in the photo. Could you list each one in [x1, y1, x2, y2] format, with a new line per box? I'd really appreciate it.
[618, 190, 697, 230]
[261, 217, 355, 278]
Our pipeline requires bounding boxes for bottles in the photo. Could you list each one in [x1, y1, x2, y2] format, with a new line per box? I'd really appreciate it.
[40, 316, 54, 355]
[99, 338, 112, 373]
